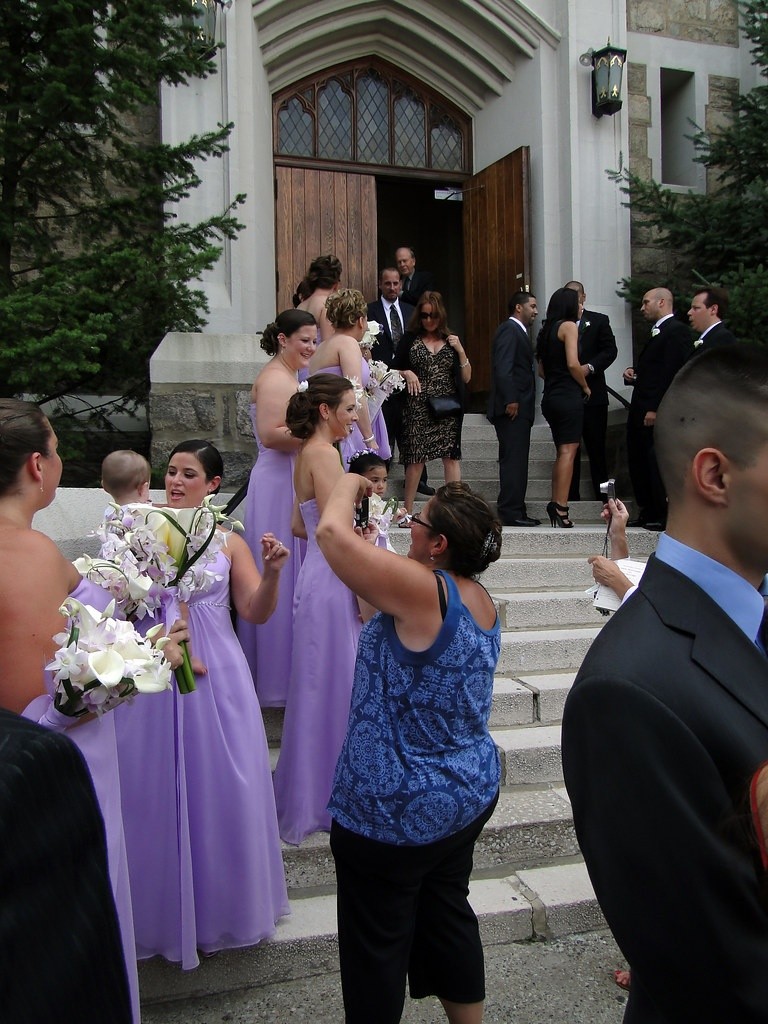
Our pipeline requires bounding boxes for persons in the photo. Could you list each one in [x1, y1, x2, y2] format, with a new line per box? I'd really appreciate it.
[316, 474, 503, 1024]
[561, 336, 768, 1024]
[366, 268, 437, 496]
[564, 281, 617, 500]
[588, 496, 639, 991]
[0, 252, 406, 1024]
[680, 287, 738, 369]
[485, 288, 544, 528]
[394, 248, 438, 306]
[536, 288, 591, 528]
[624, 287, 690, 532]
[391, 290, 472, 527]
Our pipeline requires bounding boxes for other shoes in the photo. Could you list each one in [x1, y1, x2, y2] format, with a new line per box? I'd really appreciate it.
[397, 514, 415, 528]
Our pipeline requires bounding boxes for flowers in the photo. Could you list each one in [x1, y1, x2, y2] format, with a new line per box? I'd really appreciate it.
[580, 320, 591, 336]
[44, 494, 245, 723]
[358, 320, 384, 350]
[368, 496, 399, 525]
[648, 328, 661, 339]
[693, 340, 704, 350]
[297, 379, 309, 392]
[344, 359, 406, 411]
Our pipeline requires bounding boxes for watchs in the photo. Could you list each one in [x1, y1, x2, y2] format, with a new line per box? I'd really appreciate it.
[588, 363, 595, 374]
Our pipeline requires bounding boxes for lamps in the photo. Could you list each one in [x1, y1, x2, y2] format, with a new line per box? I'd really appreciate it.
[181, 0, 233, 48]
[580, 47, 594, 66]
[592, 37, 627, 118]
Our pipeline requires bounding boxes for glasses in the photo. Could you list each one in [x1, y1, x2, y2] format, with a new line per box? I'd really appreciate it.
[409, 512, 439, 535]
[421, 311, 442, 320]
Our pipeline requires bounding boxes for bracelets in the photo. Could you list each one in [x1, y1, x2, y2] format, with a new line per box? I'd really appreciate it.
[362, 434, 375, 443]
[459, 359, 469, 369]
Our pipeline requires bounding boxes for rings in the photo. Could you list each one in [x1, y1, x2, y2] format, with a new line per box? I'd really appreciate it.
[279, 541, 282, 546]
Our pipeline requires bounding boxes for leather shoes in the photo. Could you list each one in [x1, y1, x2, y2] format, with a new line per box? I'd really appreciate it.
[626, 518, 645, 526]
[644, 522, 666, 532]
[502, 514, 543, 526]
[402, 478, 436, 496]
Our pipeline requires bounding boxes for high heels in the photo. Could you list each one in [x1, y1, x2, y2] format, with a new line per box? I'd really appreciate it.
[547, 500, 574, 527]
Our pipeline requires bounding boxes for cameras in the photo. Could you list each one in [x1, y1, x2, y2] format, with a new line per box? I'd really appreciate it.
[600, 478, 616, 502]
[356, 497, 369, 531]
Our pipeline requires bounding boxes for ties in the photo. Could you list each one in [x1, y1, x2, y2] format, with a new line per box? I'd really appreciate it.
[402, 275, 410, 297]
[652, 325, 656, 333]
[390, 304, 404, 352]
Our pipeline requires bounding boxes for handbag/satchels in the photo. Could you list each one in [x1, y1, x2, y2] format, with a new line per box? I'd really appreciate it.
[425, 396, 462, 419]
